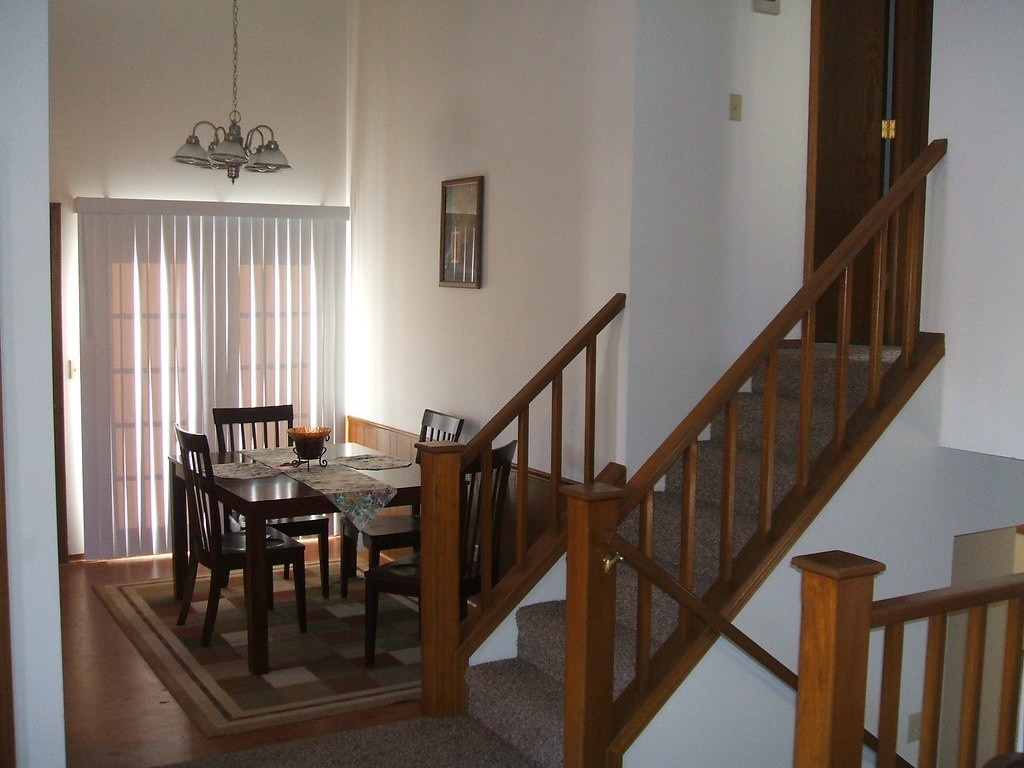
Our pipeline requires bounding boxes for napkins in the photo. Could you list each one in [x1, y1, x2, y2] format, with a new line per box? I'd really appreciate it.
[333, 455, 412, 471]
[202, 464, 281, 480]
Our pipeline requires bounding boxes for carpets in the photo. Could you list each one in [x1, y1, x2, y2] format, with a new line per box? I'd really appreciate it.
[93, 558, 422, 738]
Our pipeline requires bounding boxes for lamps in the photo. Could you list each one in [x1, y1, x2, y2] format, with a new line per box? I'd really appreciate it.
[170, 0, 292, 185]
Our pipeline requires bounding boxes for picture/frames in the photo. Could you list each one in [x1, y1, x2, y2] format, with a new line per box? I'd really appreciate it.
[438, 176, 485, 289]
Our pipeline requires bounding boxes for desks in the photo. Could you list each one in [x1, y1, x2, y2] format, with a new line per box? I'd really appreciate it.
[166, 442, 421, 675]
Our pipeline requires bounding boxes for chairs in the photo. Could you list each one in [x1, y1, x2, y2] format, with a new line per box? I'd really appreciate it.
[339, 408, 465, 599]
[174, 422, 308, 646]
[361, 438, 518, 669]
[212, 403, 329, 609]
